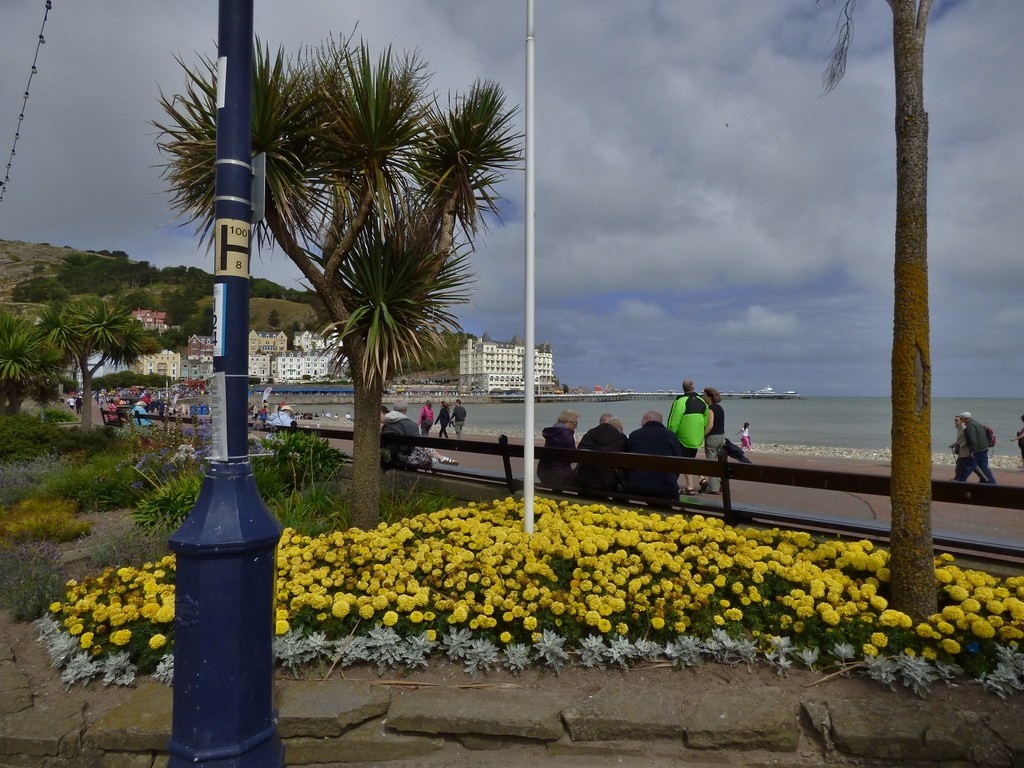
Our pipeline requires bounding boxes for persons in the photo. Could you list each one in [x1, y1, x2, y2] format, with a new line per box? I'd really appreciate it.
[251, 403, 351, 427]
[434, 402, 450, 438]
[451, 399, 466, 439]
[1011, 415, 1024, 467]
[668, 380, 708, 495]
[131, 401, 153, 427]
[107, 399, 127, 423]
[67, 397, 82, 414]
[380, 401, 459, 466]
[537, 409, 682, 501]
[951, 412, 996, 483]
[93, 388, 200, 404]
[418, 400, 434, 435]
[704, 387, 725, 495]
[736, 423, 753, 451]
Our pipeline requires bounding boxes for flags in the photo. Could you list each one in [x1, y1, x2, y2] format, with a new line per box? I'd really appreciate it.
[263, 388, 272, 403]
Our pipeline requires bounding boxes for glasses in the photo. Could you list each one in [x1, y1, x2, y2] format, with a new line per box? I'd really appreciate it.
[954, 418, 959, 421]
[568, 420, 578, 426]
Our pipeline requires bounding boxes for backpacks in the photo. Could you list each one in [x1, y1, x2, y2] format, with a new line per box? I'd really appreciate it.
[981, 422, 996, 447]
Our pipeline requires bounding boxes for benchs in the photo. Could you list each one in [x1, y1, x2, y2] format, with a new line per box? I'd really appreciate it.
[100, 407, 1024, 564]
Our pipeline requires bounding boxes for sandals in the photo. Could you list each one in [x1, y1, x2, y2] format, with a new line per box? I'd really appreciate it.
[698, 479, 708, 494]
[678, 487, 696, 496]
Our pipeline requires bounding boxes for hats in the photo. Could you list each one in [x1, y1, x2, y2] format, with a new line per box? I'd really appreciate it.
[280, 405, 294, 417]
[960, 411, 971, 419]
[136, 401, 148, 406]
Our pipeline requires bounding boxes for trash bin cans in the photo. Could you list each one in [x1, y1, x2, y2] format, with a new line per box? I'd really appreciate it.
[190, 404, 208, 415]
